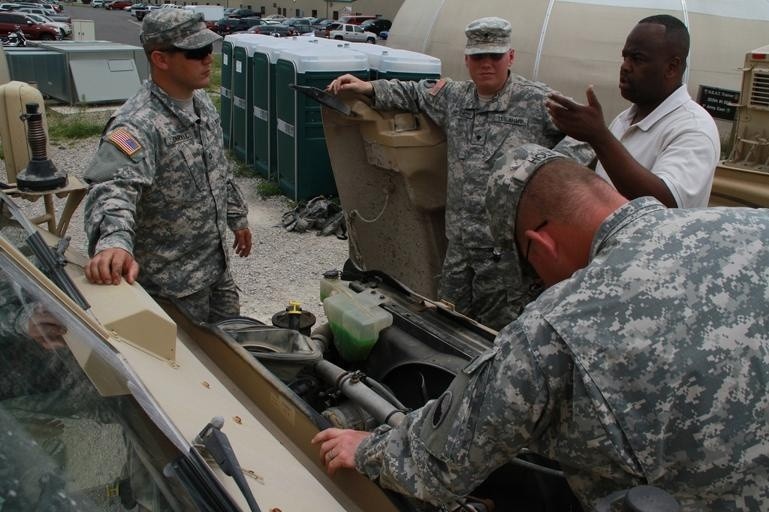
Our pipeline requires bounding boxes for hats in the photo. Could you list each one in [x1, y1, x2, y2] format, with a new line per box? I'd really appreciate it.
[465, 16, 512, 54]
[140, 7, 223, 50]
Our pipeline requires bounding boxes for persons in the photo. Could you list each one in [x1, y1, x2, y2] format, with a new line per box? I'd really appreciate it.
[83, 4, 254, 329]
[324, 18, 599, 333]
[0, 195, 87, 398]
[539, 13, 723, 211]
[310, 150, 769, 512]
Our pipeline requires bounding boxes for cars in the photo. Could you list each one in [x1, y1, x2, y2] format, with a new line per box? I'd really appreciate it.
[80, 0, 393, 45]
[1, 0, 74, 41]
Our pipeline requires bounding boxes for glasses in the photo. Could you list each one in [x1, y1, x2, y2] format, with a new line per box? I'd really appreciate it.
[518, 219, 550, 280]
[469, 50, 510, 62]
[149, 42, 213, 61]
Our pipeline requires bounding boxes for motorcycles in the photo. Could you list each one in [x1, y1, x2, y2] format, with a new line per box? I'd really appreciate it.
[1, 24, 27, 47]
